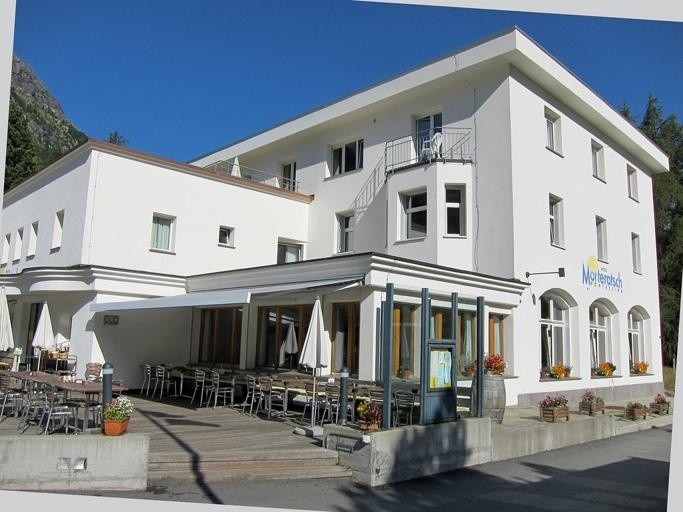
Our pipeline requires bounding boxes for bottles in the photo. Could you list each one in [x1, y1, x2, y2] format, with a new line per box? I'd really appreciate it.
[61, 374, 76, 383]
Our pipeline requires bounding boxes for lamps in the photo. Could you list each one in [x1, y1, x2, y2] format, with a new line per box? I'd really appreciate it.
[526, 267, 565, 278]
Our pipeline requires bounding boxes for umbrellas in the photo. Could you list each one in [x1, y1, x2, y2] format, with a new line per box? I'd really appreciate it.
[300, 294, 329, 427]
[32, 301, 56, 372]
[284, 317, 298, 368]
[0, 284, 14, 351]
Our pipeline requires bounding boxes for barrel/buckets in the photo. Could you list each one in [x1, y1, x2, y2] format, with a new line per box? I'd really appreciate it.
[469, 375, 506, 423]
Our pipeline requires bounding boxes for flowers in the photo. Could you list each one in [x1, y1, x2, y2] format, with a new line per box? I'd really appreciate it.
[464, 353, 649, 380]
[581, 389, 605, 404]
[626, 401, 645, 409]
[654, 394, 672, 409]
[539, 393, 568, 409]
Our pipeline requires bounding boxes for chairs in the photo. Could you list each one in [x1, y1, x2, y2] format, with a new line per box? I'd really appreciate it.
[420, 132, 443, 160]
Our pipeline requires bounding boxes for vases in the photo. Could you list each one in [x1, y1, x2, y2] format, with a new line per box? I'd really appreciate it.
[540, 407, 570, 422]
[625, 409, 647, 420]
[650, 403, 669, 415]
[579, 402, 606, 416]
